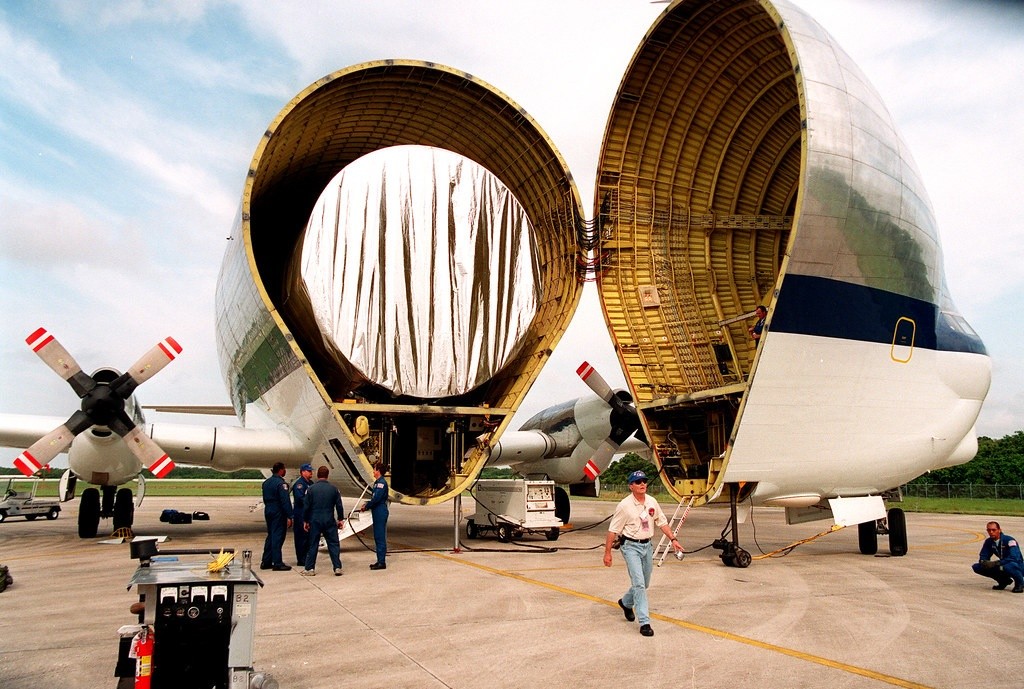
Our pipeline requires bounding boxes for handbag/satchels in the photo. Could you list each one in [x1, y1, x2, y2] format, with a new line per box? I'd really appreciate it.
[159, 507, 210, 524]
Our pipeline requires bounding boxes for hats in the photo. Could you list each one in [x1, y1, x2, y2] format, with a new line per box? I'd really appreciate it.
[300, 464, 314, 470]
[628, 470, 649, 484]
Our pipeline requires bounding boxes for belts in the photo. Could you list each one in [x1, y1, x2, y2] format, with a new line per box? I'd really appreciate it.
[622, 534, 651, 543]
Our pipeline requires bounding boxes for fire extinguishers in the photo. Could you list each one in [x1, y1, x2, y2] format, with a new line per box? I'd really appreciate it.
[133, 628, 154, 689]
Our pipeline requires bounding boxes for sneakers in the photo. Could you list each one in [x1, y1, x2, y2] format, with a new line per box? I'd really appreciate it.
[640, 624, 654, 636]
[618, 599, 635, 621]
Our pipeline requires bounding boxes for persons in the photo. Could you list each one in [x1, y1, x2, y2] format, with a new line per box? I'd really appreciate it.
[972, 522, 1024, 593]
[361, 463, 389, 571]
[749, 305, 768, 348]
[260, 462, 345, 576]
[604, 470, 686, 636]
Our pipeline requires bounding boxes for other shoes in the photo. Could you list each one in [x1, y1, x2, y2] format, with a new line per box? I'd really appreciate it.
[260, 563, 272, 569]
[370, 562, 386, 570]
[334, 568, 342, 575]
[992, 579, 1013, 590]
[1012, 581, 1024, 593]
[296, 561, 305, 566]
[300, 569, 315, 576]
[273, 564, 292, 570]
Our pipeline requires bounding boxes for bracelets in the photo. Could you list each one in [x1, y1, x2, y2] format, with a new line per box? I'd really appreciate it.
[671, 537, 677, 542]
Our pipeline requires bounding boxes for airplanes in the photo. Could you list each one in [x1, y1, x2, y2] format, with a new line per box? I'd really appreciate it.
[0, 1, 994, 565]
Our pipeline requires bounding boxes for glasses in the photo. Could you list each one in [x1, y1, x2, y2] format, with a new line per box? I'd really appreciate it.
[632, 479, 647, 484]
[987, 528, 999, 532]
